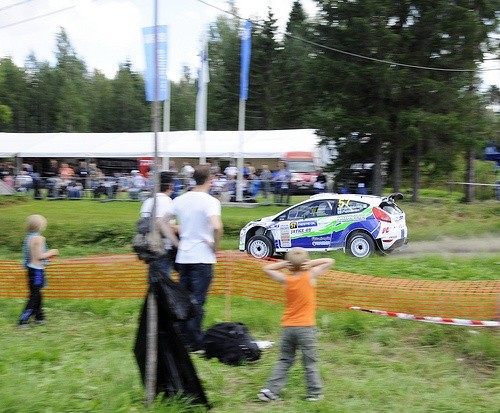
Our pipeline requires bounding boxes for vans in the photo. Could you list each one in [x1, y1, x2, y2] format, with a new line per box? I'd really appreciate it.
[279, 151, 321, 194]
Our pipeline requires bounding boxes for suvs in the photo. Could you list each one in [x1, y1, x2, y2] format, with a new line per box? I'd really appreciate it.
[333, 162, 377, 195]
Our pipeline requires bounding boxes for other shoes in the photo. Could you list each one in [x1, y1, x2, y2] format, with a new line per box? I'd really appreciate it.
[257, 387, 284, 402]
[17, 324, 30, 329]
[305, 394, 324, 401]
[35, 320, 45, 325]
[185, 344, 205, 353]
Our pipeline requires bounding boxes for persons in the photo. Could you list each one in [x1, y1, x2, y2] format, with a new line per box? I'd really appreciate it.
[17, 214, 60, 330]
[135, 166, 223, 354]
[2, 160, 367, 206]
[256, 247, 336, 403]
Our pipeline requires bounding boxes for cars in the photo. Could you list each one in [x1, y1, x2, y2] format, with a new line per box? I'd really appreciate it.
[237, 192, 410, 258]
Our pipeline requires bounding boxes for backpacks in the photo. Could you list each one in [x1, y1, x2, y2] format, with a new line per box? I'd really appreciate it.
[200, 323, 261, 365]
[132, 211, 168, 264]
